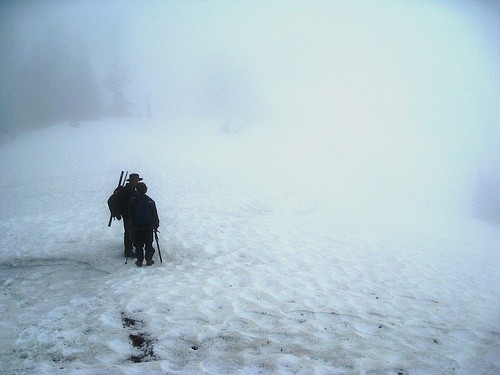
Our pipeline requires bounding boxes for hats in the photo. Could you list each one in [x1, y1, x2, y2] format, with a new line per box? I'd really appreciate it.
[126, 173, 143, 181]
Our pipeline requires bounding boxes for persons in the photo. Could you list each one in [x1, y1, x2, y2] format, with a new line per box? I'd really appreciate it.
[122, 173, 159, 267]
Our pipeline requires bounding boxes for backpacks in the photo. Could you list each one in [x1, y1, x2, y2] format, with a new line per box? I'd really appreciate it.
[133, 196, 152, 226]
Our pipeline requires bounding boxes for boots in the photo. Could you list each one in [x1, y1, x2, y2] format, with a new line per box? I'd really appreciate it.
[145, 247, 155, 266]
[135, 248, 144, 266]
[124, 236, 135, 257]
